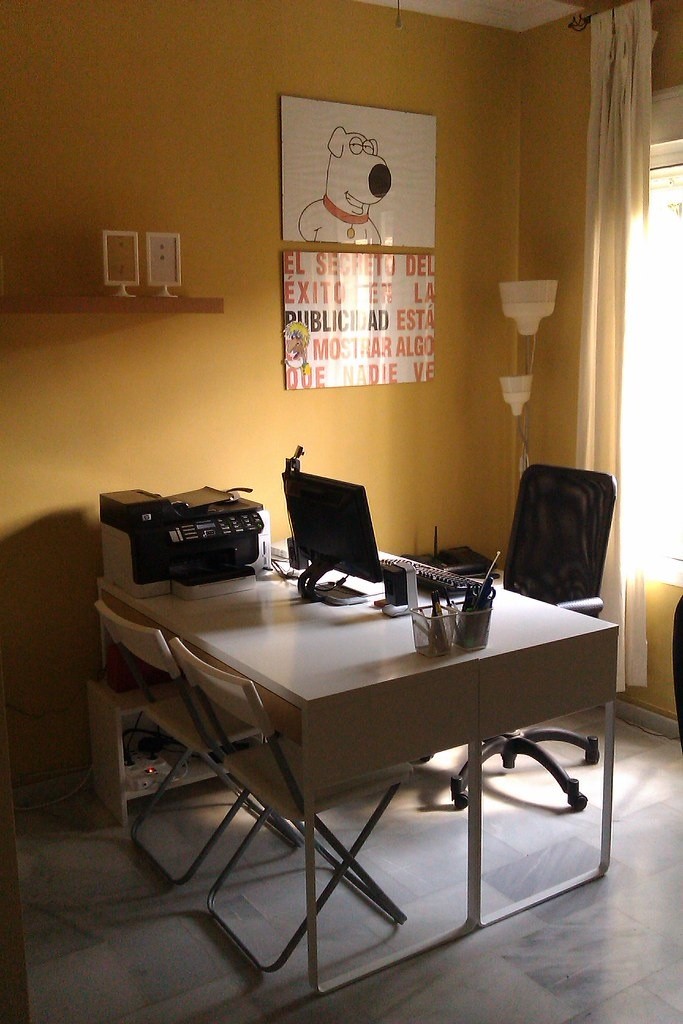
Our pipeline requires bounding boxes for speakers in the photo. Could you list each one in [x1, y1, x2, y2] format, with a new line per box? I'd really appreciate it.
[374, 562, 418, 616]
[286, 537, 309, 578]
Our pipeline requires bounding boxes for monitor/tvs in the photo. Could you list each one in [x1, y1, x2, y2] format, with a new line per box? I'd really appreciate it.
[281, 471, 384, 606]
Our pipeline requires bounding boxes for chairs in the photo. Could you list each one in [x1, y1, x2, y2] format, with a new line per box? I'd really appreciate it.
[407, 463, 618, 811]
[95, 599, 304, 886]
[168, 636, 407, 974]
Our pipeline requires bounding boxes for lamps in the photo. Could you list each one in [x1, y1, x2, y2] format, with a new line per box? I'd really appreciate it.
[497, 278, 559, 480]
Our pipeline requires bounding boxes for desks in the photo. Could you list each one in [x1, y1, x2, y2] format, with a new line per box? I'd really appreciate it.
[96, 540, 619, 997]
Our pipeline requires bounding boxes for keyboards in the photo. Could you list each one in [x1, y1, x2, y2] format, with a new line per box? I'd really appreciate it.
[380, 559, 481, 592]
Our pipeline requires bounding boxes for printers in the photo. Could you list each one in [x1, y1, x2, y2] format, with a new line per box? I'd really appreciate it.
[99, 486, 264, 601]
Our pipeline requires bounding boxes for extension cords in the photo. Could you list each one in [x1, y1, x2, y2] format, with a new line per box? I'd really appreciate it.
[124, 750, 175, 790]
